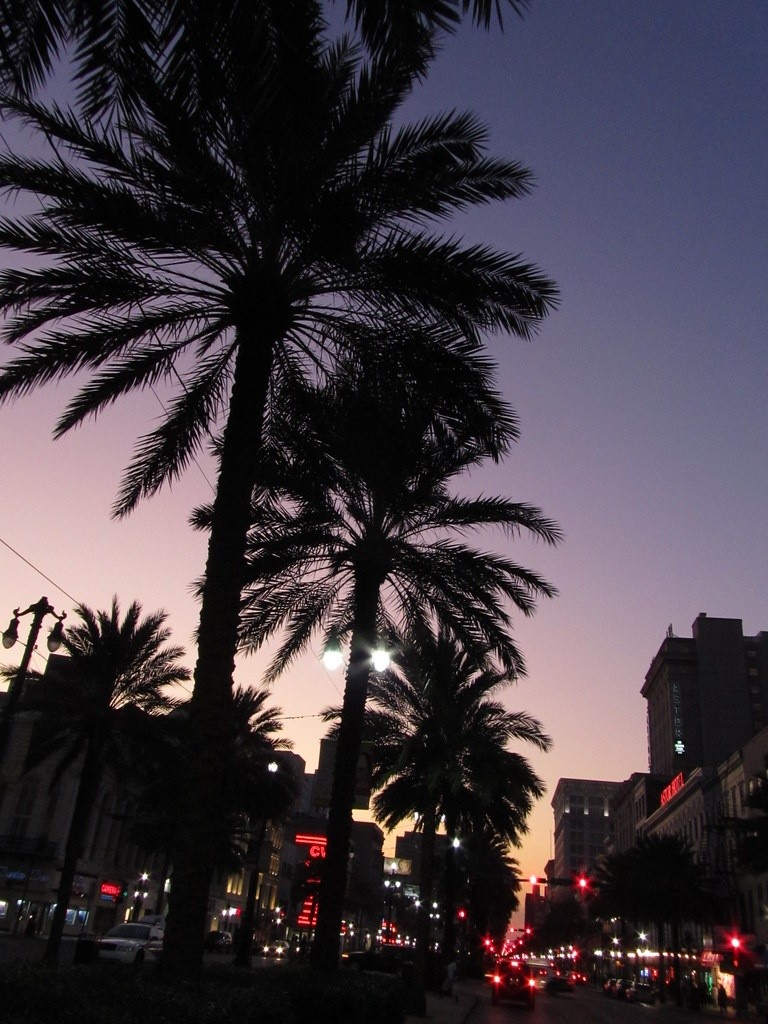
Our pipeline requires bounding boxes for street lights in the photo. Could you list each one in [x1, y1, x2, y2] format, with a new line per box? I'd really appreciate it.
[0, 597, 68, 764]
[503, 931, 648, 989]
[221, 900, 237, 930]
[130, 869, 150, 921]
[384, 862, 401, 945]
[270, 903, 281, 939]
[428, 895, 440, 954]
[340, 919, 441, 951]
[325, 621, 392, 673]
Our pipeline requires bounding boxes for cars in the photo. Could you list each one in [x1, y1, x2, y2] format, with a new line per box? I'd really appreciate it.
[262, 941, 302, 956]
[207, 929, 233, 944]
[95, 923, 165, 965]
[494, 956, 655, 1009]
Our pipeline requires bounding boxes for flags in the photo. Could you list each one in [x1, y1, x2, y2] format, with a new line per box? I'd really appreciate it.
[352, 742, 374, 811]
[313, 739, 336, 806]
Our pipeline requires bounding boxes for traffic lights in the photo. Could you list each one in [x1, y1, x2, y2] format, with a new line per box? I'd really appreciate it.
[577, 877, 597, 888]
[549, 878, 573, 885]
[530, 877, 546, 883]
[510, 927, 531, 934]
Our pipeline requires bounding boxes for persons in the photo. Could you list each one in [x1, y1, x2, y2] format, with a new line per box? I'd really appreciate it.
[711, 984, 718, 1009]
[718, 985, 727, 1014]
[396, 951, 413, 981]
[439, 960, 460, 1003]
[287, 936, 298, 962]
[648, 976, 707, 1005]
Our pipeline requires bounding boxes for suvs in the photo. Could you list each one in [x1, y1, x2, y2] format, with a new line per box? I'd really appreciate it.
[348, 942, 417, 974]
